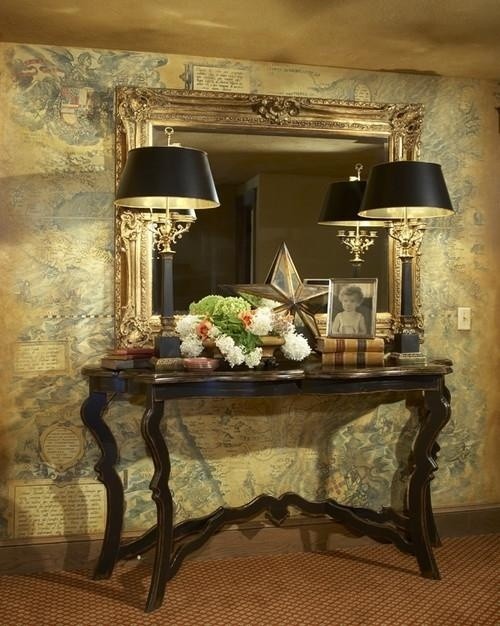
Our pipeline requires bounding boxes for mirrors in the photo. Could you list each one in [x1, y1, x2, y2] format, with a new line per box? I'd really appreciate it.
[114, 84, 424, 347]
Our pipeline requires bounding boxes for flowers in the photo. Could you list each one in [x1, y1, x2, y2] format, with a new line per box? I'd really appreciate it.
[189, 295, 224, 316]
[175, 295, 311, 368]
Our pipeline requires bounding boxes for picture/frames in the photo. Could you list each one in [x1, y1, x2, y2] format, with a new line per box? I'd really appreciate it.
[327, 278, 378, 340]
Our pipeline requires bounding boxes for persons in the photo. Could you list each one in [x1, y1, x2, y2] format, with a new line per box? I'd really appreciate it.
[333, 286, 367, 334]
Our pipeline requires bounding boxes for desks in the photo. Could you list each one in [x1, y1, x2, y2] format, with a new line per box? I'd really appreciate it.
[80, 360, 451, 613]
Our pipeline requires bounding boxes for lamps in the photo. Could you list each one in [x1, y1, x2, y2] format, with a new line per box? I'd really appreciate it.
[316, 162, 392, 277]
[357, 160, 455, 368]
[114, 128, 221, 372]
[116, 209, 197, 229]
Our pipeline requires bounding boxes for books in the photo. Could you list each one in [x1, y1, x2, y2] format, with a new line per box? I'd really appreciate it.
[315, 337, 385, 366]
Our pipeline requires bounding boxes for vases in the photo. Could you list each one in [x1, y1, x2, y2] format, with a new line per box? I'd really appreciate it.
[203, 336, 286, 370]
